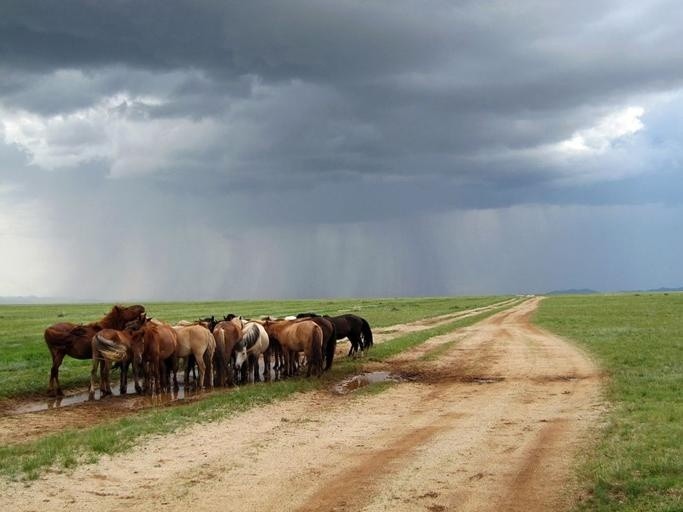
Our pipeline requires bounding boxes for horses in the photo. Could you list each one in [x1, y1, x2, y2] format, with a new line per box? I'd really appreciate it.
[44, 303, 373, 395]
